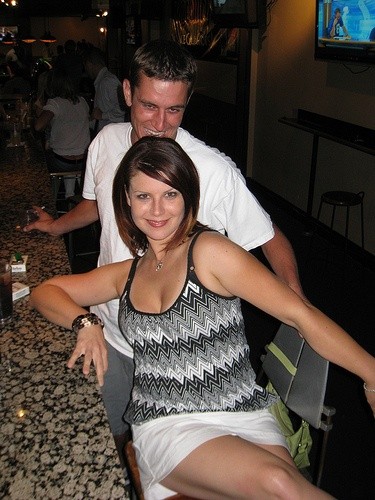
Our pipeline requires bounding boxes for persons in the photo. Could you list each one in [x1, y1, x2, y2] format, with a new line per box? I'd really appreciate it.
[82, 47, 126, 130]
[33, 69, 90, 199]
[30, 136, 375, 500]
[327, 8, 348, 40]
[24, 40, 308, 435]
[4, 36, 76, 104]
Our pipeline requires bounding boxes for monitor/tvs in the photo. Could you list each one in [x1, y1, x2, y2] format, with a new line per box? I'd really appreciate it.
[212, 0, 267, 30]
[315, 0, 375, 65]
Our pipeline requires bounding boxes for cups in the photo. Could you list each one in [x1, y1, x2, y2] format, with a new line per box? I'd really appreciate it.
[0, 263, 13, 324]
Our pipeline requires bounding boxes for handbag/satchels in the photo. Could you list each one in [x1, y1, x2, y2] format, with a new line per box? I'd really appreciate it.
[266, 343, 312, 470]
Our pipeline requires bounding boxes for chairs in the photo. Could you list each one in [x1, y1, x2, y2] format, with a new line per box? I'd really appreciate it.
[125, 324, 336, 500]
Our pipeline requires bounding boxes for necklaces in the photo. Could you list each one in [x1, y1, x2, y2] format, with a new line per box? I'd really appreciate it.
[152, 250, 167, 271]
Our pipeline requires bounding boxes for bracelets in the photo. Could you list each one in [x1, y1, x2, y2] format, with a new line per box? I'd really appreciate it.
[364, 383, 375, 392]
[72, 313, 104, 335]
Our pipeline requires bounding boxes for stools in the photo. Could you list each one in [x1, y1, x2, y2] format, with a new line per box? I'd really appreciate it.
[51, 171, 83, 213]
[315, 190, 365, 248]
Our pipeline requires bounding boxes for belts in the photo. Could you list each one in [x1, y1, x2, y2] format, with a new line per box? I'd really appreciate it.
[53, 152, 86, 164]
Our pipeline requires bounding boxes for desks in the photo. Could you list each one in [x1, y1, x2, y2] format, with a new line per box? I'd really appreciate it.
[279, 109, 375, 214]
